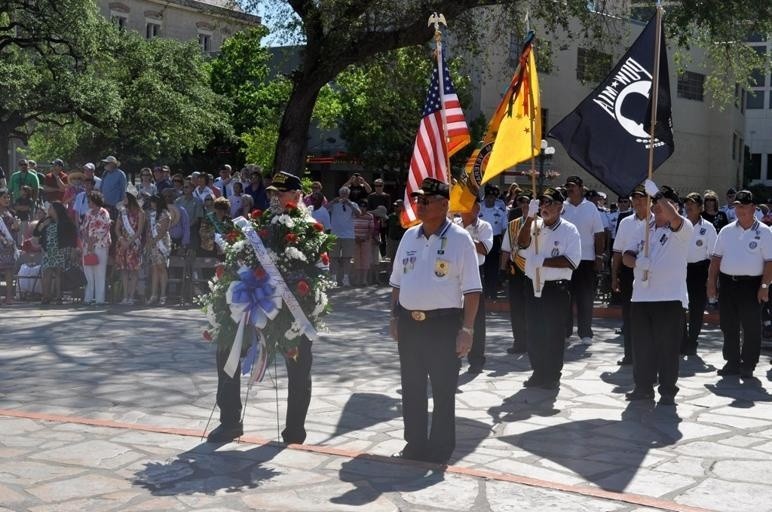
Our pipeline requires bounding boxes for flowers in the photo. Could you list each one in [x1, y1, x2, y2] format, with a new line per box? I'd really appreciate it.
[185, 196, 339, 391]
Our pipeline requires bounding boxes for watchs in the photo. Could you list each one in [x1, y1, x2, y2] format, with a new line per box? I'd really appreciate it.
[462, 326, 475, 336]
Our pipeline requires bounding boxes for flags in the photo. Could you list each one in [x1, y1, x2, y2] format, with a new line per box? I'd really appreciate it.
[446, 34, 542, 211]
[398, 41, 470, 226]
[546, 9, 677, 193]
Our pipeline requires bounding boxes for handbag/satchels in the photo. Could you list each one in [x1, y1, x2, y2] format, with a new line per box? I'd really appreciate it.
[83, 252, 99, 266]
[60, 267, 88, 291]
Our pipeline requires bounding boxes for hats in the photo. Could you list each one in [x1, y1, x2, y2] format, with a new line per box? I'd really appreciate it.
[49, 156, 118, 169]
[369, 207, 389, 221]
[265, 170, 302, 191]
[629, 184, 755, 205]
[410, 177, 450, 200]
[393, 198, 404, 206]
[352, 172, 361, 176]
[161, 164, 170, 172]
[484, 176, 608, 202]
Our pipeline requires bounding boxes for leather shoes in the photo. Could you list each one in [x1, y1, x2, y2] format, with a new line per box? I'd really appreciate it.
[717, 362, 753, 377]
[660, 395, 673, 405]
[206, 424, 244, 445]
[617, 357, 633, 363]
[390, 446, 453, 464]
[626, 390, 654, 399]
[507, 346, 525, 354]
[524, 376, 559, 388]
[468, 366, 482, 373]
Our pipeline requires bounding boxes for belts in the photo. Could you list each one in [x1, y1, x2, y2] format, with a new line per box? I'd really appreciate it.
[396, 303, 463, 322]
[719, 274, 763, 282]
[525, 276, 568, 287]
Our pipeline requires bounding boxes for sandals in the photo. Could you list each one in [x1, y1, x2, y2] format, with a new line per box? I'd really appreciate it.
[40, 294, 167, 307]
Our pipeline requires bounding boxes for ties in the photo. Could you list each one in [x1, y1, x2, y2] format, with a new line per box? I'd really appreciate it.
[223, 185, 226, 198]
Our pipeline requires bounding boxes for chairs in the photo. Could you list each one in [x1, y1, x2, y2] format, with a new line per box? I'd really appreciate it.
[164, 256, 185, 307]
[110, 258, 151, 302]
[185, 257, 222, 311]
[10, 250, 58, 304]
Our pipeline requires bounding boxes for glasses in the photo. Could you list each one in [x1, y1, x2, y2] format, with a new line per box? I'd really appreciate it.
[20, 161, 36, 167]
[142, 174, 150, 176]
[173, 180, 181, 182]
[342, 204, 346, 211]
[413, 197, 445, 207]
[619, 200, 628, 203]
[539, 200, 558, 207]
[266, 190, 294, 199]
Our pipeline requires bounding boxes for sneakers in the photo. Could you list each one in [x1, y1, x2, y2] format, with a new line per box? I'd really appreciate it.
[563, 334, 593, 346]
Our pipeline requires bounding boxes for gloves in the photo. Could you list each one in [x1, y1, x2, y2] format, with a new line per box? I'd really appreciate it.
[635, 257, 649, 269]
[528, 198, 539, 219]
[524, 256, 545, 272]
[644, 177, 660, 197]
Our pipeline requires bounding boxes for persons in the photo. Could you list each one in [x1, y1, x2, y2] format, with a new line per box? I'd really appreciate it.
[389, 177, 483, 464]
[453, 196, 493, 376]
[1, 157, 270, 308]
[476, 176, 772, 405]
[204, 170, 325, 444]
[304, 173, 405, 287]
